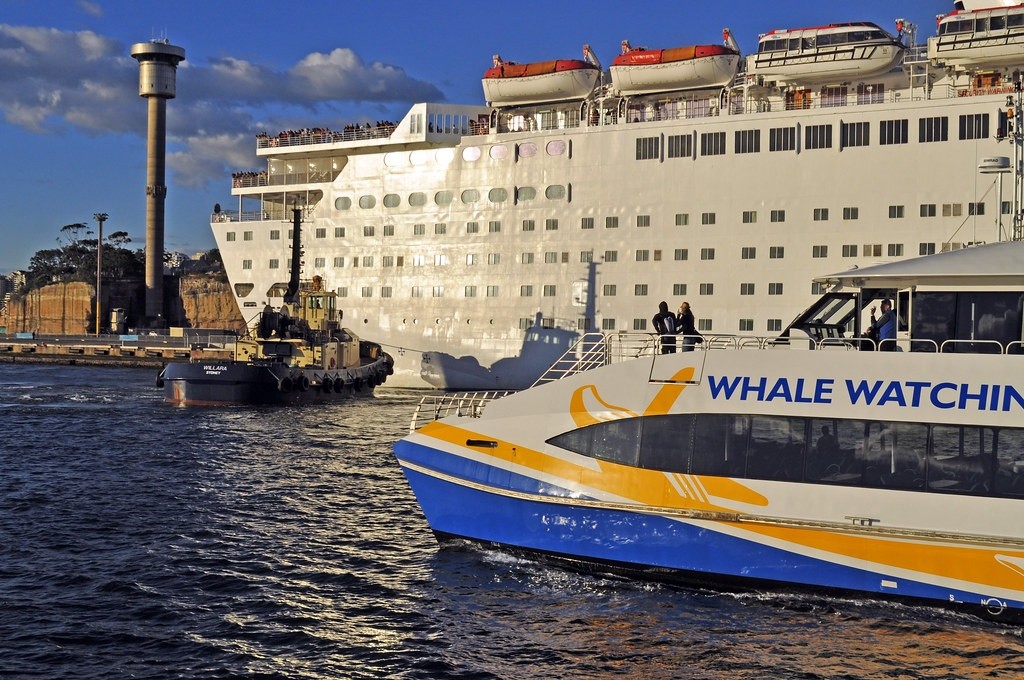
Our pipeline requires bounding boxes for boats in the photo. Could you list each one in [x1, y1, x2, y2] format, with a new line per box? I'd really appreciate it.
[609, 26, 742, 99]
[927, 1, 1024, 71]
[392, 69, 1024, 619]
[480, 42, 603, 107]
[755, 20, 912, 89]
[158, 201, 395, 416]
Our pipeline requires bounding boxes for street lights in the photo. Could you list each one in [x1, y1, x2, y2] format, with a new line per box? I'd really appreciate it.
[91, 212, 109, 337]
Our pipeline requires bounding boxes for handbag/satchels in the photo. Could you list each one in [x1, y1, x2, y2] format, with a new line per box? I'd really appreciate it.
[693, 329, 703, 343]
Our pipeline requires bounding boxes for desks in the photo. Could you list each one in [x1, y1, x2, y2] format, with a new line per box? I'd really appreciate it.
[929, 480, 959, 488]
[821, 473, 862, 481]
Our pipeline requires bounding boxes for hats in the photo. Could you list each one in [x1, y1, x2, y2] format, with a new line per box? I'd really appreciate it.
[881, 299, 892, 304]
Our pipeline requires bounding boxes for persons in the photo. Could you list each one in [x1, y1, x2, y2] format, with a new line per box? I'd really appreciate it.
[870, 299, 897, 351]
[652, 300, 677, 354]
[818, 426, 838, 473]
[675, 302, 696, 352]
[230, 119, 460, 187]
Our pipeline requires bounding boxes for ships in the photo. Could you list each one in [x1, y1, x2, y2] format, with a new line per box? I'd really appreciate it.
[208, 1, 1024, 396]
